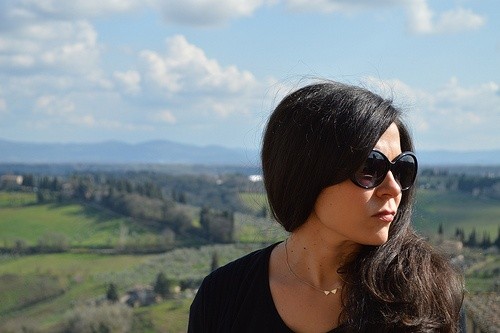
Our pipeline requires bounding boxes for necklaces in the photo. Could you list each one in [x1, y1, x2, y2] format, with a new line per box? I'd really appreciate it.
[285, 237, 342, 296]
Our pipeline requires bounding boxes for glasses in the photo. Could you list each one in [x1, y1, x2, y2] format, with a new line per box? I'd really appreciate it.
[351, 149, 419, 191]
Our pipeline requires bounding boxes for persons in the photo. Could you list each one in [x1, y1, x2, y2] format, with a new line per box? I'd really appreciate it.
[185, 73, 468, 333]
